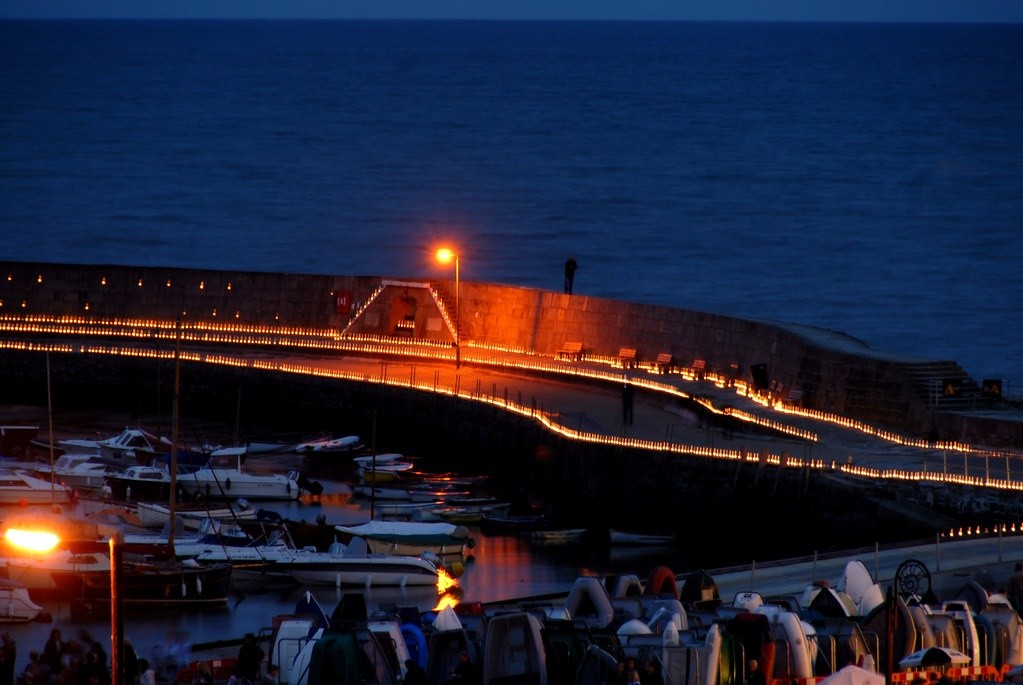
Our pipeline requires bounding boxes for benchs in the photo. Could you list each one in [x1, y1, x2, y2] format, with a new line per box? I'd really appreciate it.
[651, 354, 674, 374]
[687, 360, 705, 380]
[618, 348, 637, 369]
[556, 342, 582, 363]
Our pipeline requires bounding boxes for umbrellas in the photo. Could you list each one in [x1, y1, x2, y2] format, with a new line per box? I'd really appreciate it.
[816, 647, 971, 685]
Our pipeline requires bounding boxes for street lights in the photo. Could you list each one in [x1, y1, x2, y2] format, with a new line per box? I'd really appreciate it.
[438, 248, 460, 370]
[2, 517, 125, 685]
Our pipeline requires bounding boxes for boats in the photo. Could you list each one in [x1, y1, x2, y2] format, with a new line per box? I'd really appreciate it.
[1, 308, 1023, 685]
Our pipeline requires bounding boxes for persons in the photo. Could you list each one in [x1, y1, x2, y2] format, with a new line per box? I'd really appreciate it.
[0, 625, 288, 685]
[613, 659, 666, 685]
[402, 652, 479, 685]
[746, 660, 766, 685]
[622, 382, 634, 427]
